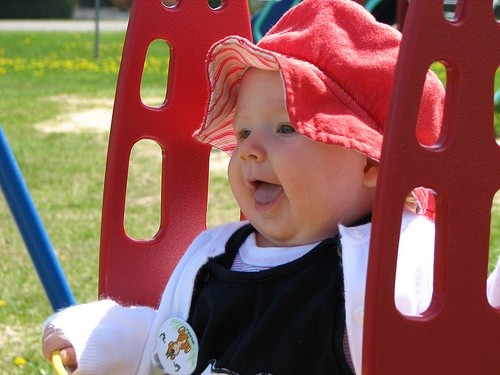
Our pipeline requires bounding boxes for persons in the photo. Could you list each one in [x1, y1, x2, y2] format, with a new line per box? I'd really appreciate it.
[40, 0, 500, 375]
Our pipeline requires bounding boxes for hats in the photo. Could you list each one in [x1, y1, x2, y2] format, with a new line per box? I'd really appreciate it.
[190, 2, 449, 219]
